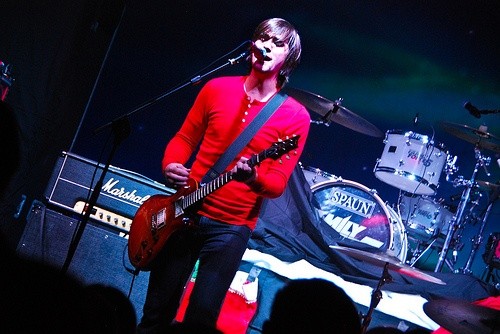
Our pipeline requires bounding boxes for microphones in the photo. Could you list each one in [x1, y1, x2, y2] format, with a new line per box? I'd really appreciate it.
[248, 40, 266, 60]
[465, 102, 481, 119]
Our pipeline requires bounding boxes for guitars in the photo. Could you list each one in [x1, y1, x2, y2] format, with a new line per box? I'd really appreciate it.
[128, 134, 301, 270]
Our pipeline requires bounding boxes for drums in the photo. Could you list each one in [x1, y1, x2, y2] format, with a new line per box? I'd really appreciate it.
[298, 164, 343, 188]
[373, 129, 449, 196]
[309, 176, 408, 265]
[396, 190, 462, 250]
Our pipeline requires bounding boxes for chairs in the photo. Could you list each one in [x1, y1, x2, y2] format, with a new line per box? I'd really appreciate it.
[482, 231, 500, 285]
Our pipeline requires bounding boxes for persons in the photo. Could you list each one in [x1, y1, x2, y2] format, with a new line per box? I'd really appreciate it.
[0, 256, 361, 334]
[136, 18, 311, 334]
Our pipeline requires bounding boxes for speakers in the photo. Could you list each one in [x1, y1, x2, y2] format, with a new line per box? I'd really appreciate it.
[15, 203, 151, 327]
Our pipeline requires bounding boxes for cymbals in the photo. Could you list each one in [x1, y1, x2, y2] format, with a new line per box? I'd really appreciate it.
[475, 180, 500, 204]
[280, 85, 383, 138]
[436, 118, 500, 152]
[325, 239, 449, 288]
